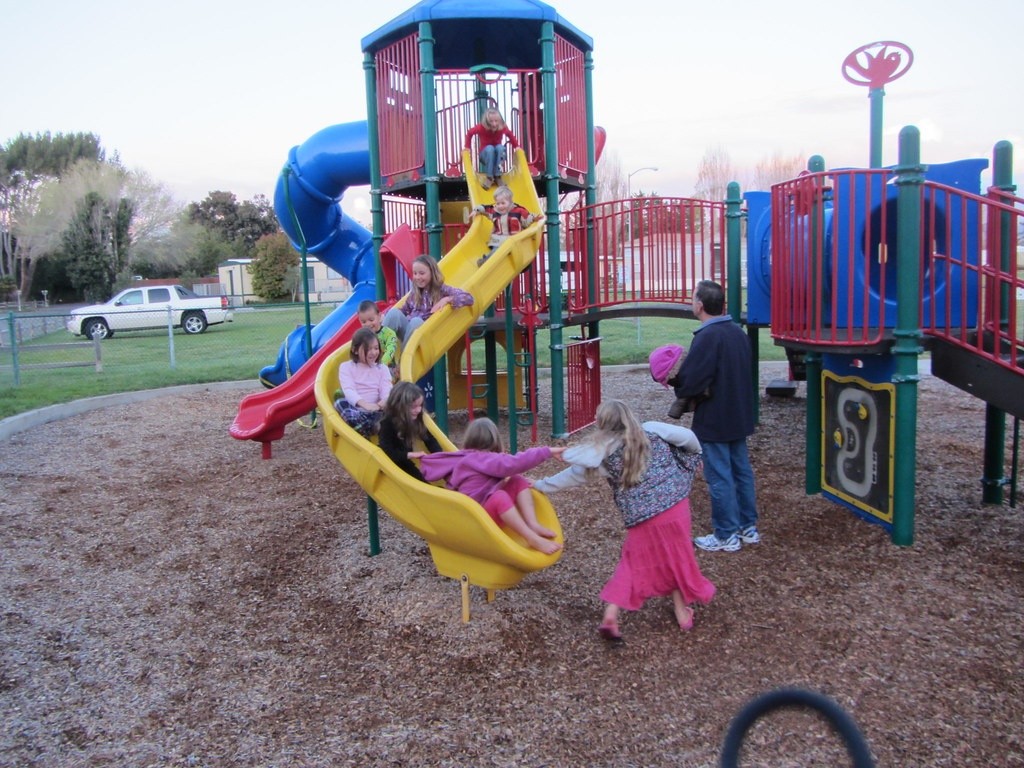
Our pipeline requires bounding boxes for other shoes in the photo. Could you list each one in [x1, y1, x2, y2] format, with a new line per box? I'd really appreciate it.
[494, 179, 506, 187]
[482, 179, 492, 188]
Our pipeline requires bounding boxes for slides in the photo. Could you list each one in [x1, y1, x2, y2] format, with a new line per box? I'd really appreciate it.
[314, 145, 566, 591]
[257, 108, 426, 390]
[229, 222, 424, 443]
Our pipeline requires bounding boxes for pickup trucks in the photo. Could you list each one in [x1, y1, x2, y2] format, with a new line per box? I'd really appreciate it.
[67, 284, 234, 341]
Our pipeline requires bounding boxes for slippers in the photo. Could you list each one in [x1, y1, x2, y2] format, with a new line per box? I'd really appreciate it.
[598, 626, 621, 638]
[680, 607, 693, 629]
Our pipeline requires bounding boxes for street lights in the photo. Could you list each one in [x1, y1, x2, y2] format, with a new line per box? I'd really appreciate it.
[627, 166, 658, 244]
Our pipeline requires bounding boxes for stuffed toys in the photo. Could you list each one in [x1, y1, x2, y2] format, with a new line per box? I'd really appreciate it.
[649, 344, 710, 419]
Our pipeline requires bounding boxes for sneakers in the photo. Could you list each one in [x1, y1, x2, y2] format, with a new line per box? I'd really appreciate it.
[737, 526, 759, 544]
[694, 532, 740, 552]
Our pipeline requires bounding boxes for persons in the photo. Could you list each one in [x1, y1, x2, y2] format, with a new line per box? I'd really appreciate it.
[675, 281, 761, 552]
[420, 418, 569, 555]
[468, 186, 543, 268]
[465, 108, 521, 190]
[338, 329, 395, 433]
[358, 300, 399, 384]
[384, 254, 473, 351]
[526, 401, 716, 640]
[378, 379, 444, 486]
